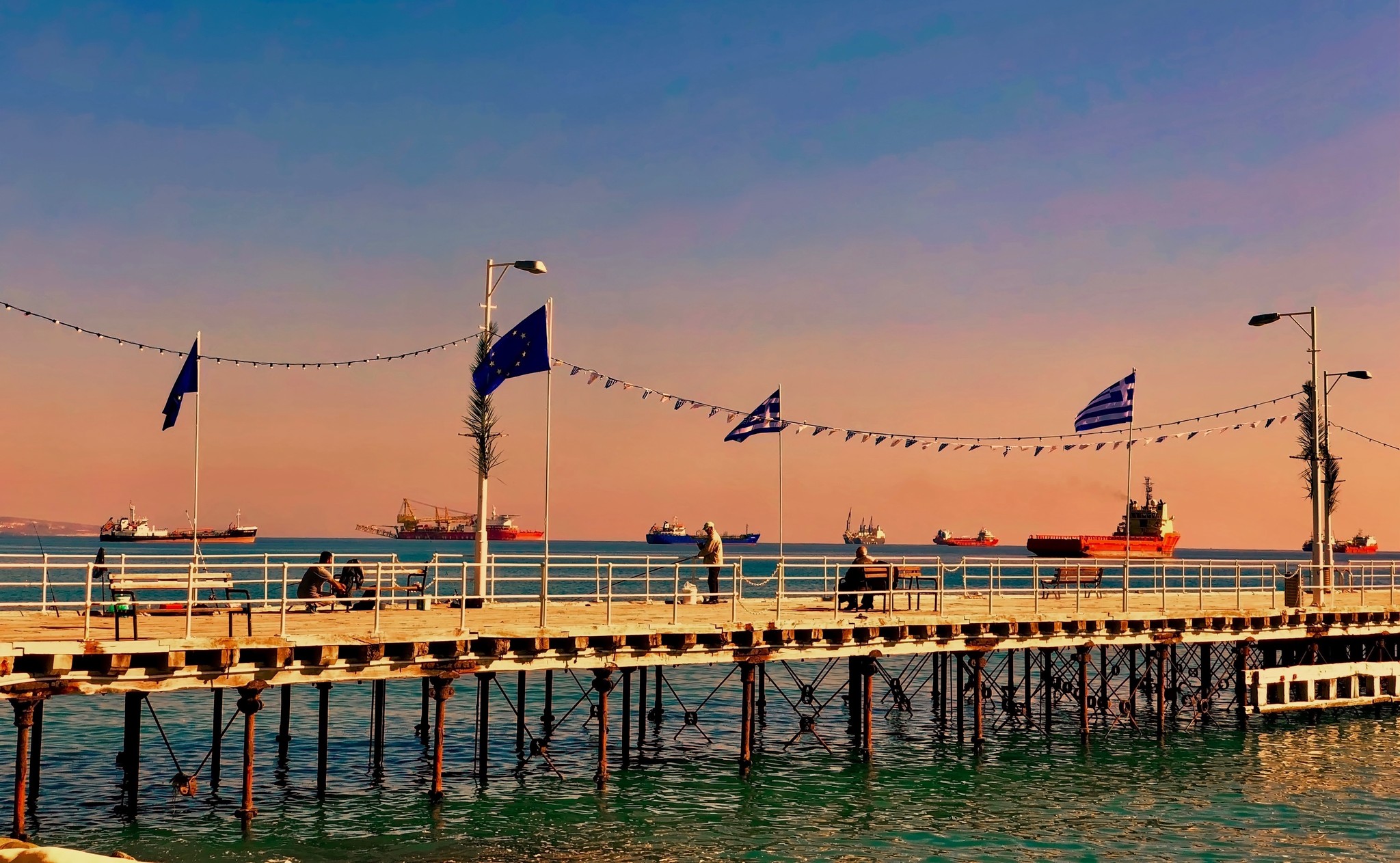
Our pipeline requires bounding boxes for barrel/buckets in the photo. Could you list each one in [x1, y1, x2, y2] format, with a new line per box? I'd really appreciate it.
[682, 589, 697, 605]
[416, 594, 431, 610]
[109, 595, 130, 610]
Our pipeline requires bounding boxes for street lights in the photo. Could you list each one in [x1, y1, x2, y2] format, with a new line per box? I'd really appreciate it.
[475, 256, 546, 603]
[1247, 307, 1324, 607]
[1324, 371, 1372, 589]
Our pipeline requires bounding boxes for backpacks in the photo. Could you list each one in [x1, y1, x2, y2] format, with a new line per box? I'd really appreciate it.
[869, 561, 899, 589]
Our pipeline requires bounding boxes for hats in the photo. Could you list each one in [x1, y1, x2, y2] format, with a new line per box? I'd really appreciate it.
[702, 521, 714, 530]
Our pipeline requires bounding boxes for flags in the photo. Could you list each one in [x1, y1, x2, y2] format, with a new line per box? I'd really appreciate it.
[1074, 367, 1136, 432]
[473, 304, 551, 398]
[554, 360, 737, 422]
[781, 410, 1304, 462]
[162, 331, 201, 434]
[724, 389, 781, 443]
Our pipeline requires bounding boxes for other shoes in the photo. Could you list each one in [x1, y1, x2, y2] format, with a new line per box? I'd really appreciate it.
[858, 603, 874, 610]
[305, 604, 320, 614]
[705, 599, 719, 605]
[844, 604, 856, 611]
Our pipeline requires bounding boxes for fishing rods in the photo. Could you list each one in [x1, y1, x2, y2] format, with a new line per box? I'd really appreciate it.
[570, 555, 699, 602]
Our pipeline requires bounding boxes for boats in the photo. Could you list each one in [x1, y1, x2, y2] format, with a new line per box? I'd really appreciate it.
[646, 516, 761, 545]
[100, 500, 257, 543]
[1302, 528, 1378, 554]
[355, 499, 544, 541]
[932, 526, 998, 546]
[843, 507, 885, 545]
[1026, 477, 1181, 558]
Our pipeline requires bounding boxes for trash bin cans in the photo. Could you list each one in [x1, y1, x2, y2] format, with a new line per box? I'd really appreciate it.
[1285, 571, 1304, 608]
[1323, 567, 1330, 593]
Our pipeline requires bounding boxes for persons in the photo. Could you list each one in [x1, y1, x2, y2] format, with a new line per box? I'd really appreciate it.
[834, 547, 874, 610]
[697, 522, 723, 604]
[297, 552, 345, 613]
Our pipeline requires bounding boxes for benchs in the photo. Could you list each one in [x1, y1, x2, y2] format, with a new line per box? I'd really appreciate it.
[329, 560, 432, 614]
[1039, 566, 1106, 601]
[107, 569, 253, 640]
[837, 566, 923, 613]
